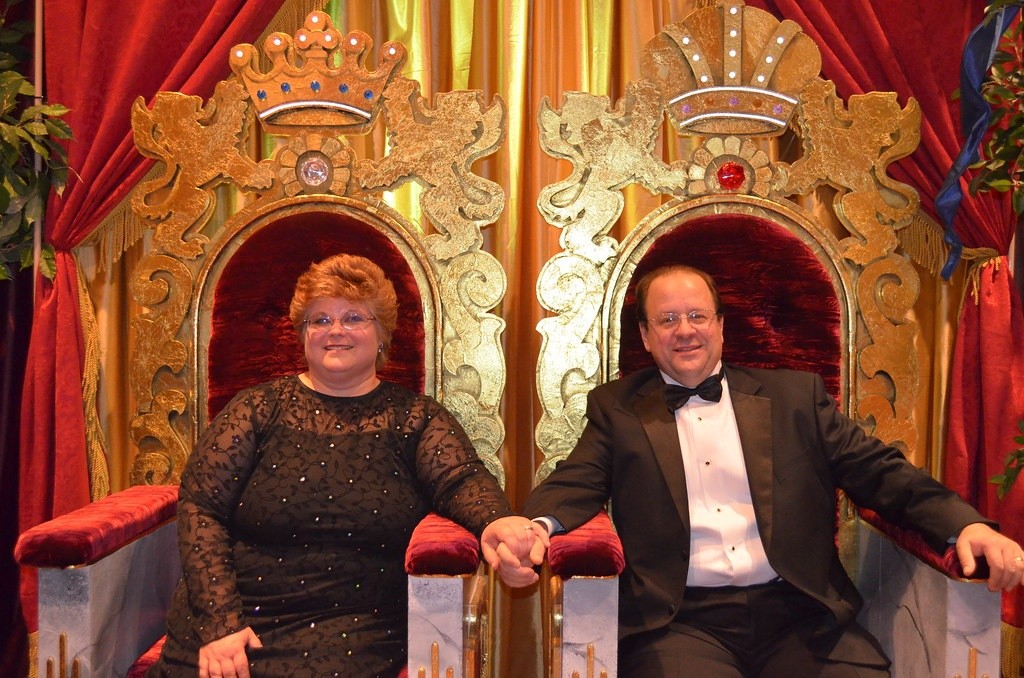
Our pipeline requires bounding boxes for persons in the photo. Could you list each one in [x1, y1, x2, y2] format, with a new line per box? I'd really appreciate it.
[496, 262, 1024, 678]
[141, 253, 553, 678]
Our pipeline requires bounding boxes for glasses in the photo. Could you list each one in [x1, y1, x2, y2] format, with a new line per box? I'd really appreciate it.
[646, 309, 721, 329]
[302, 310, 378, 331]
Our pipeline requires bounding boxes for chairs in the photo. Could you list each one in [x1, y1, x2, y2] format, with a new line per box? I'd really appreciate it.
[535, 3, 1012, 677]
[10, 11, 509, 675]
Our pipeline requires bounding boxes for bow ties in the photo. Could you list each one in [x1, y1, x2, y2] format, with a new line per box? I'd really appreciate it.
[663, 374, 723, 413]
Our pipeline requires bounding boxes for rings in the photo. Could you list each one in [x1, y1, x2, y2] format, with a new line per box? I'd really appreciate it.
[1013, 556, 1024, 564]
[525, 526, 534, 532]
